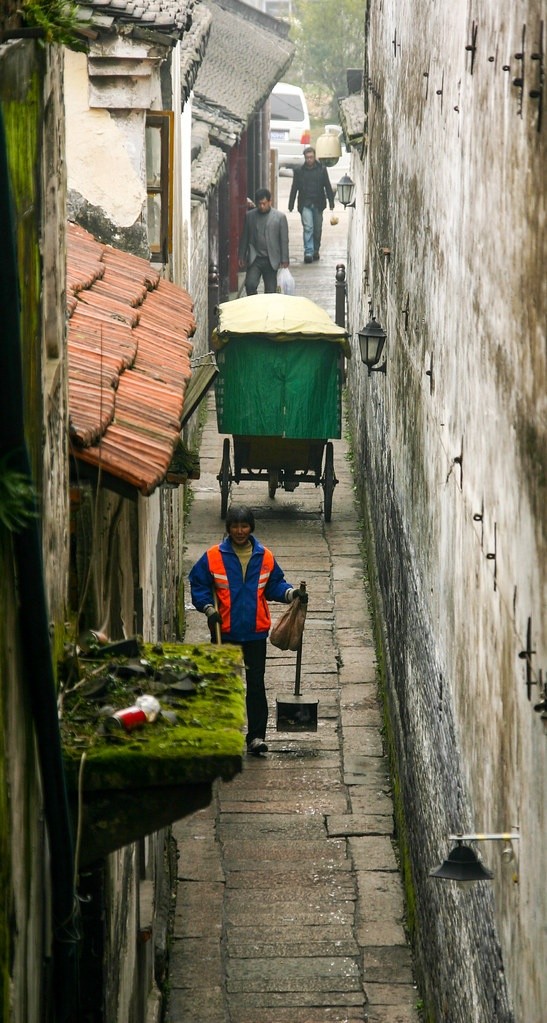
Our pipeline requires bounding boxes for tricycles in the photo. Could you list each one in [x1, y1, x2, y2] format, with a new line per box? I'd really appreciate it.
[216, 292, 354, 522]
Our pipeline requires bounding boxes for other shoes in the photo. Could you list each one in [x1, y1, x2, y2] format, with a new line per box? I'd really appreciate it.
[305, 254, 313, 263]
[313, 252, 319, 260]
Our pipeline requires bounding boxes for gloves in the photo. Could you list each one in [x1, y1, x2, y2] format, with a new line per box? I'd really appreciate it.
[285, 588, 308, 604]
[202, 604, 222, 639]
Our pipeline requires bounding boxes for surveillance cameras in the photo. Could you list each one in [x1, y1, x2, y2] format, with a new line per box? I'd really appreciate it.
[316, 134, 342, 167]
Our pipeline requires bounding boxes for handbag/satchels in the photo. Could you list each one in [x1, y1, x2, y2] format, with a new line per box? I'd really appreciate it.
[276, 267, 295, 296]
[270, 595, 308, 651]
[330, 209, 338, 225]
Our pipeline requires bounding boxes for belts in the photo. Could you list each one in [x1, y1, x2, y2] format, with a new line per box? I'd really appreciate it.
[256, 256, 269, 259]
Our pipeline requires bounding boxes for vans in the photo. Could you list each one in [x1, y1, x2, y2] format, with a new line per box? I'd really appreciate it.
[270, 81, 311, 171]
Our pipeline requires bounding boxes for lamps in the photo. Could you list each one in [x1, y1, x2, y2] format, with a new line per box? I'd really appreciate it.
[337, 173, 356, 211]
[429, 832, 521, 881]
[355, 317, 388, 377]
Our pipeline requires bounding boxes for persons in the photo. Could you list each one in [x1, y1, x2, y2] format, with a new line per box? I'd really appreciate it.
[188, 505, 309, 754]
[239, 189, 290, 296]
[288, 147, 335, 262]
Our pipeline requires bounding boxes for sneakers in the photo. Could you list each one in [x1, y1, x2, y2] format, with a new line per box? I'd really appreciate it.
[247, 737, 268, 752]
